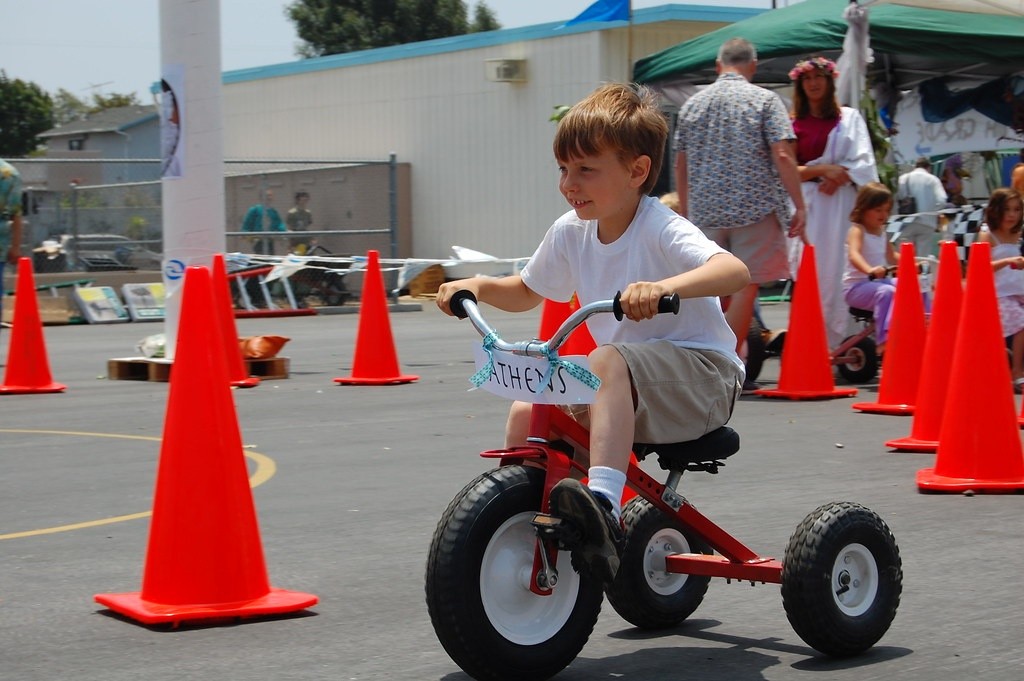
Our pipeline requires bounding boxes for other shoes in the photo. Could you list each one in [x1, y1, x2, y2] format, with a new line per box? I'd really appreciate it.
[743, 379, 759, 390]
[1012, 377, 1024, 390]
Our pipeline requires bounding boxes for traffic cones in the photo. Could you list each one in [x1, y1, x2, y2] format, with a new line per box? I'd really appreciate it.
[0, 257, 68, 394]
[885, 241, 964, 450]
[332, 250, 419, 386]
[539, 293, 597, 357]
[214, 254, 261, 388]
[852, 244, 927, 414]
[92, 265, 319, 628]
[916, 241, 1024, 493]
[753, 245, 858, 399]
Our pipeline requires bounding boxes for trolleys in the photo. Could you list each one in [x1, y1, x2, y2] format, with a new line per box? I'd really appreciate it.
[278, 246, 354, 305]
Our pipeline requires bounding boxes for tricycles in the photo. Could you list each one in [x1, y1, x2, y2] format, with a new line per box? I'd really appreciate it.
[738, 315, 781, 389]
[828, 260, 932, 384]
[425, 289, 903, 680]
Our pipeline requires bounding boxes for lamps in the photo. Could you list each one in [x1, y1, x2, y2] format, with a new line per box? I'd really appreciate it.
[69, 133, 89, 150]
[484, 59, 529, 83]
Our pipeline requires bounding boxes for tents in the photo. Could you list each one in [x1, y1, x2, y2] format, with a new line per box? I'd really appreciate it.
[630, 0, 1024, 104]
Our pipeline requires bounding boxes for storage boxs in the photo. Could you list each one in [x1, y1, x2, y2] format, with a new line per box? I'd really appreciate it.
[120, 282, 166, 323]
[409, 263, 445, 298]
[74, 286, 130, 325]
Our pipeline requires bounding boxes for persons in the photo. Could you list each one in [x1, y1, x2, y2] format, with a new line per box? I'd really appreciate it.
[432, 81, 753, 589]
[841, 181, 935, 357]
[670, 35, 811, 397]
[0, 157, 19, 333]
[785, 54, 884, 380]
[241, 187, 292, 307]
[942, 153, 973, 205]
[899, 156, 947, 212]
[975, 187, 1024, 396]
[285, 190, 318, 309]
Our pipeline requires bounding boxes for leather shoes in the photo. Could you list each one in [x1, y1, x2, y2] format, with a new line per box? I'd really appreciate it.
[760, 328, 788, 352]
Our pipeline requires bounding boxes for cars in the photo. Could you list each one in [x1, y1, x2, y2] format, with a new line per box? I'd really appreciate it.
[31, 235, 139, 271]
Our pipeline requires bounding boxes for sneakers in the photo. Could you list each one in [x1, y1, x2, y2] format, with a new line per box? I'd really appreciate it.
[548, 478, 628, 593]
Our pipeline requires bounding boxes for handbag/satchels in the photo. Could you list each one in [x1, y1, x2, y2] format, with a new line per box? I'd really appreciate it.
[898, 197, 916, 215]
